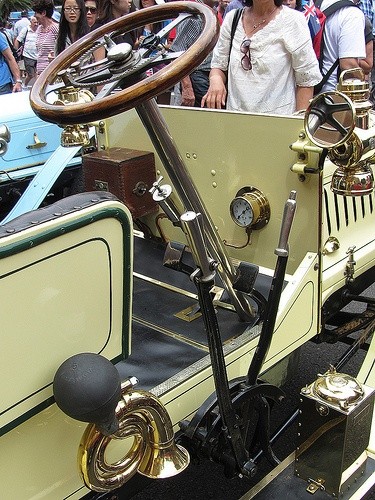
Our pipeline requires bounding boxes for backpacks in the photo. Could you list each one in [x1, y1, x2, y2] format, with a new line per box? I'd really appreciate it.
[304, 0, 361, 98]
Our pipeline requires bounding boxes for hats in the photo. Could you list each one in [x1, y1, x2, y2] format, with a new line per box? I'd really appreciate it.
[21, 12, 26, 17]
[133, 0, 165, 10]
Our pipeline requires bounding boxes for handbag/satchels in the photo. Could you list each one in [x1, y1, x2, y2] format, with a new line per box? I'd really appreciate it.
[17, 56, 26, 70]
[0, 30, 20, 63]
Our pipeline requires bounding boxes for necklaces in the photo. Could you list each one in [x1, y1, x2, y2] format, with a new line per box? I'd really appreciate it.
[251, 5, 278, 29]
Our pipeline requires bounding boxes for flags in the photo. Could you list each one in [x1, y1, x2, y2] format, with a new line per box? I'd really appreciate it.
[298, 0, 322, 40]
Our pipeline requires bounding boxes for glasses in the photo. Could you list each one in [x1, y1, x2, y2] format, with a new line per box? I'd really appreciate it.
[240, 39, 252, 71]
[85, 7, 97, 14]
[64, 6, 80, 12]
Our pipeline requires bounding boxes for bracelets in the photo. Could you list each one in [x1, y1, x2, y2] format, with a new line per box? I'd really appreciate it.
[15, 80, 23, 84]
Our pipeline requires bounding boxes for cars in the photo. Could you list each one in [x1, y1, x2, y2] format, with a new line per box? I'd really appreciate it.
[0, 0, 375, 500]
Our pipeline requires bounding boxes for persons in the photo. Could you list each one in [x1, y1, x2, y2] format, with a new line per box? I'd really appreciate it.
[31, 0, 60, 84]
[200, 0, 324, 115]
[168, 0, 246, 110]
[2, 18, 17, 29]
[13, 15, 39, 90]
[0, 17, 22, 96]
[13, 9, 32, 49]
[131, 0, 177, 106]
[281, 0, 375, 110]
[51, 0, 107, 27]
[89, 0, 146, 98]
[53, 0, 108, 97]
